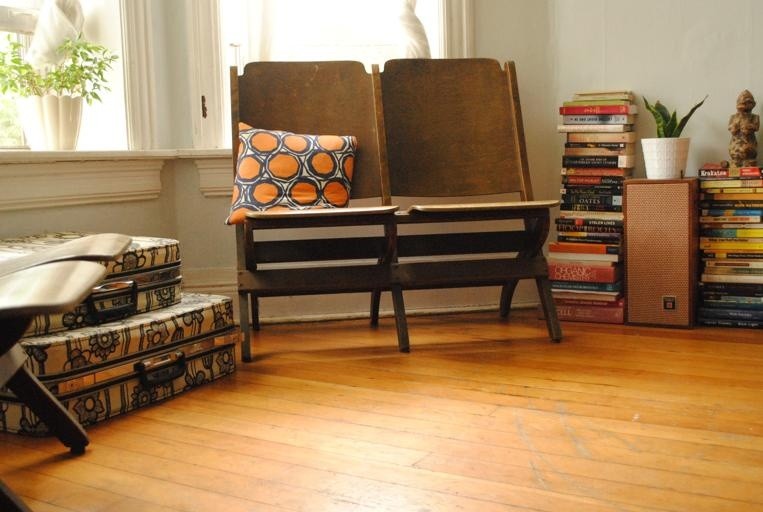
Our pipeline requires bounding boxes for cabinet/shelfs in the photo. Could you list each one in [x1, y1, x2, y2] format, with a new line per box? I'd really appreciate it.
[624, 180, 692, 327]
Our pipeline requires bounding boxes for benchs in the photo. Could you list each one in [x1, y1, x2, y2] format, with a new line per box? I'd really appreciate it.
[0, 221, 132, 512]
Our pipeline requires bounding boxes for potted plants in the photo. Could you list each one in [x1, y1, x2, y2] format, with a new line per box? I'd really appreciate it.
[640, 94, 713, 180]
[1, 31, 118, 152]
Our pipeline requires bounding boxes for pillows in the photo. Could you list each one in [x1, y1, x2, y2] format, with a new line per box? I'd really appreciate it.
[224, 122, 358, 226]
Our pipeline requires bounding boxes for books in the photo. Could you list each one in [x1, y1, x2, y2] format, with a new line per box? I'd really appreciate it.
[696, 163, 763, 329]
[535, 91, 639, 324]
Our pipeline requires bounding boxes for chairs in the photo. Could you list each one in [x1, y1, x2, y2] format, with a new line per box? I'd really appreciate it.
[230, 60, 412, 364]
[370, 57, 562, 355]
